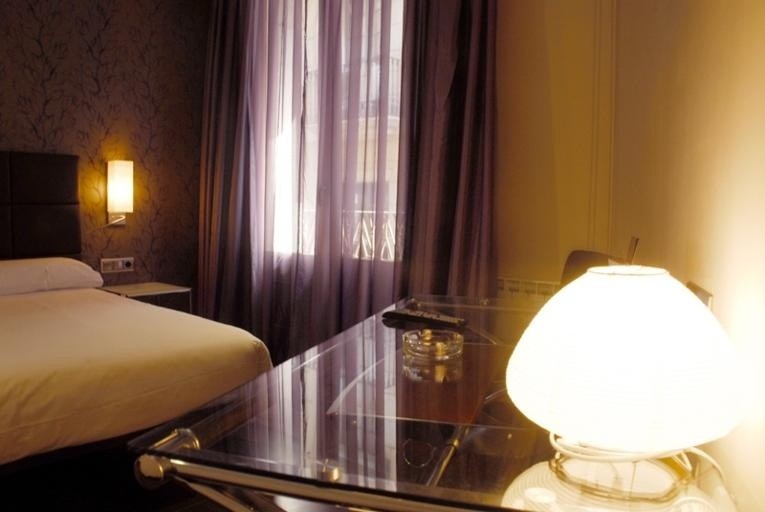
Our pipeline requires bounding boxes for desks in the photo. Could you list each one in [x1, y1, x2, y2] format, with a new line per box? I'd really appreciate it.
[130, 292, 551, 512]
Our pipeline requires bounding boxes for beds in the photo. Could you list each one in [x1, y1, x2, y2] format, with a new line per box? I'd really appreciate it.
[0, 147, 276, 512]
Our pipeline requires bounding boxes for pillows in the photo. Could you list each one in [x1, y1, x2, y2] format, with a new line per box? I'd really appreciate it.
[0, 256, 106, 297]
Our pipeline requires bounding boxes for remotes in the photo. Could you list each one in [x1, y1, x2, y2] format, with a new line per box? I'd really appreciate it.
[382, 309, 464, 327]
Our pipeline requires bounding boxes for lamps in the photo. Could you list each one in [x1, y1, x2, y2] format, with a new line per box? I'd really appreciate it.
[493, 261, 758, 512]
[104, 157, 136, 227]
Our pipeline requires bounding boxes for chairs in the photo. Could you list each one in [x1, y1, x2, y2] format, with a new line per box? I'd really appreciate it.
[558, 249, 610, 291]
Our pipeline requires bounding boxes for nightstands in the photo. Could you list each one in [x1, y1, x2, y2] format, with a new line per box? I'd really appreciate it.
[97, 282, 193, 317]
